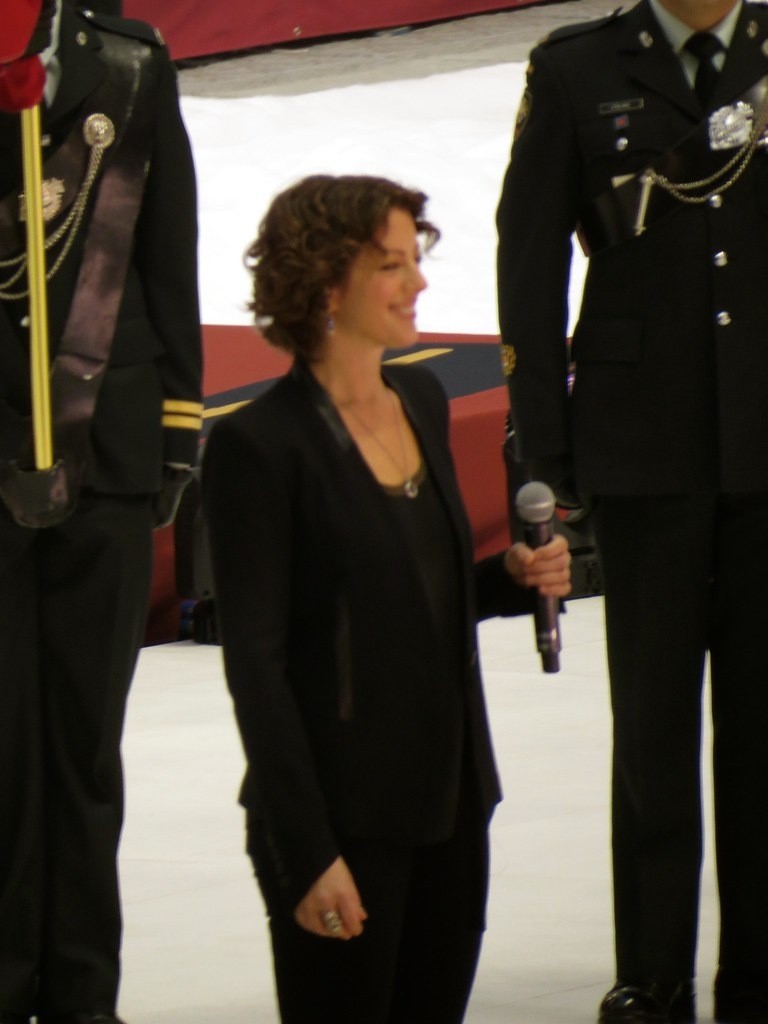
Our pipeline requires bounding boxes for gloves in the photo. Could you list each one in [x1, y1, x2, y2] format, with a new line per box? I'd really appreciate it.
[151, 466, 195, 531]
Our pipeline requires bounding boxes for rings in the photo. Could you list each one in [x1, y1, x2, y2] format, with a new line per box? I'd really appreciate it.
[323, 912, 342, 933]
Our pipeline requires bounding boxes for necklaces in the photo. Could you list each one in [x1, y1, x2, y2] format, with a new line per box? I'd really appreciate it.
[329, 387, 419, 497]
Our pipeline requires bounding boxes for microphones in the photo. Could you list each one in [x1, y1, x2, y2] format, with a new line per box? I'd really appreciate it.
[517, 481, 561, 673]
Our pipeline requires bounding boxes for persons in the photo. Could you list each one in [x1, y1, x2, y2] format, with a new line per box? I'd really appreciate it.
[498, 1, 768, 1024]
[205, 177, 572, 1024]
[2, 0, 201, 1024]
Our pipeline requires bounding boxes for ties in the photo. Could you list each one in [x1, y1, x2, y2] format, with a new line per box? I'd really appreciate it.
[685, 30, 725, 100]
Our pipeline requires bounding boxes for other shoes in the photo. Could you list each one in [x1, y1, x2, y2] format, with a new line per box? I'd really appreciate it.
[600, 973, 697, 1024]
